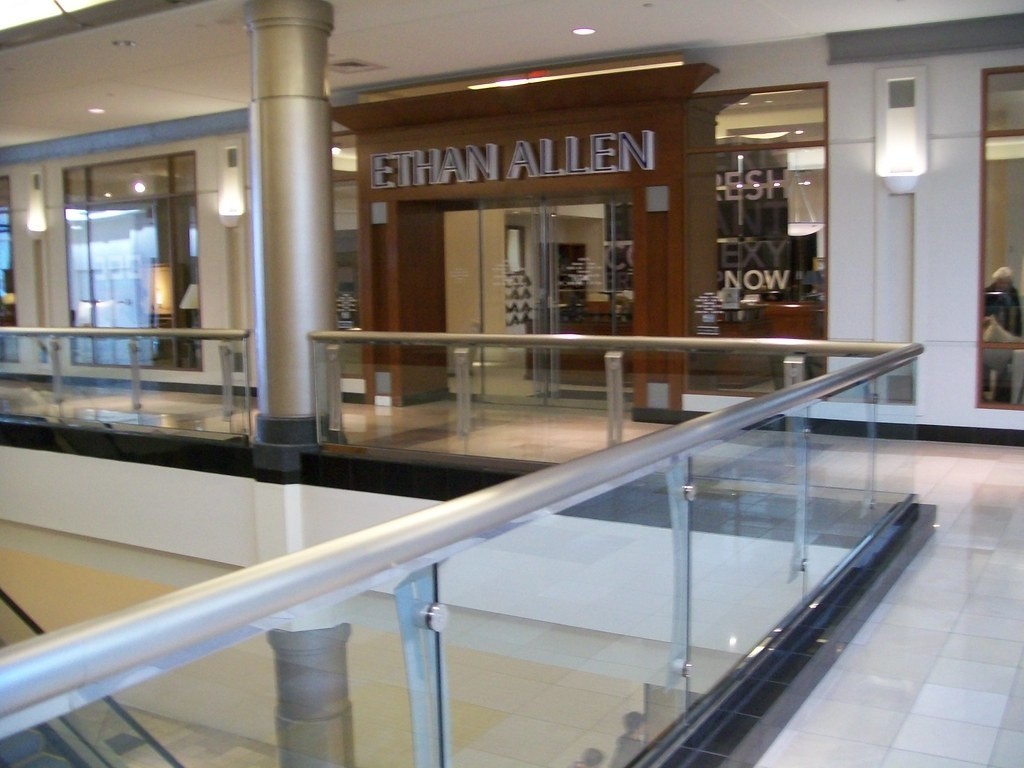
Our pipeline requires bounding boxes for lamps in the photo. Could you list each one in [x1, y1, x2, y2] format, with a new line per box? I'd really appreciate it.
[178, 283, 199, 327]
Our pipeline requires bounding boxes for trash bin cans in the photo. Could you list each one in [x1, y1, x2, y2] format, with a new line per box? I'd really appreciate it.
[769, 355, 817, 391]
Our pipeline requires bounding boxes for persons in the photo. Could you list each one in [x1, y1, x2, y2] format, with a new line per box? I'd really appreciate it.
[982, 268, 1021, 405]
[607, 711, 647, 768]
[569, 748, 603, 768]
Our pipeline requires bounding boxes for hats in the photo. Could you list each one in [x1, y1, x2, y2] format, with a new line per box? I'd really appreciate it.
[623, 711, 648, 735]
[992, 267, 1013, 278]
